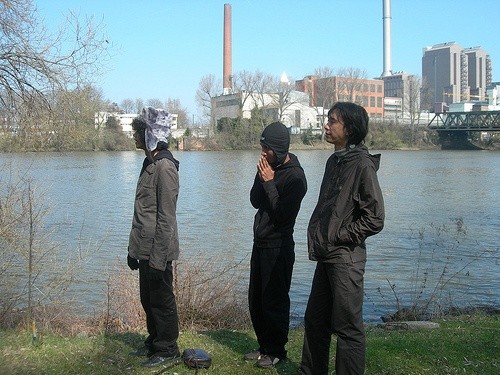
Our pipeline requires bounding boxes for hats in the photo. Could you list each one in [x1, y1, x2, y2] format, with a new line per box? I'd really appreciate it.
[259, 121, 290, 165]
[134, 105, 172, 152]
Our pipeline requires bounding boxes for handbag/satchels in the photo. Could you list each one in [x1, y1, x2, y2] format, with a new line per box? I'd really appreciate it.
[181, 349, 211, 370]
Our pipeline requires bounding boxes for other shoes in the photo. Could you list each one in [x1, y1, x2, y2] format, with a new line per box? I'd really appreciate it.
[244, 350, 264, 360]
[136, 344, 149, 356]
[253, 354, 280, 368]
[142, 352, 180, 366]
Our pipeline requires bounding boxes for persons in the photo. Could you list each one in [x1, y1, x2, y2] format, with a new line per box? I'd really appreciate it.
[300, 101, 385, 375]
[127, 106, 179, 366]
[243, 121, 307, 368]
